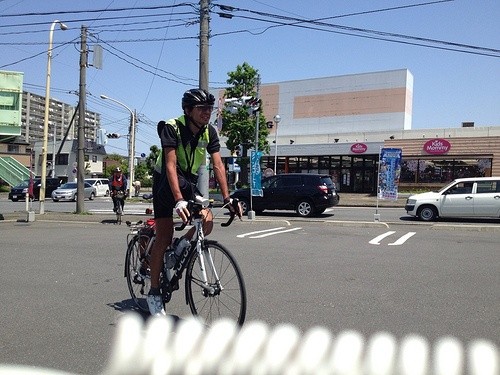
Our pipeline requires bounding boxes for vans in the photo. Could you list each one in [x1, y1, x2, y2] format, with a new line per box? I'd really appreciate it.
[83, 178, 110, 197]
[9, 178, 60, 202]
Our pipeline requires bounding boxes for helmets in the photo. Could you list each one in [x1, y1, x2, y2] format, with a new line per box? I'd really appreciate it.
[115, 167, 122, 172]
[182, 89, 215, 109]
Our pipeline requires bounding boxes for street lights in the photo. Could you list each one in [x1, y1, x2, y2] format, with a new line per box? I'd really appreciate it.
[273, 115, 281, 174]
[39, 20, 67, 214]
[100, 94, 135, 200]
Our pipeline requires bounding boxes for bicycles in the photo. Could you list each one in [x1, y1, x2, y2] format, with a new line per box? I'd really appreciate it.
[124, 197, 247, 334]
[109, 190, 128, 226]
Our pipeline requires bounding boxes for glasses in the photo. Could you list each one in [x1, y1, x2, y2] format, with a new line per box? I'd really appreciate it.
[195, 107, 213, 113]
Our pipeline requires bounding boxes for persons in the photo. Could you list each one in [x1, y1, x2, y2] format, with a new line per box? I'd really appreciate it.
[108, 166, 127, 216]
[146, 88, 243, 319]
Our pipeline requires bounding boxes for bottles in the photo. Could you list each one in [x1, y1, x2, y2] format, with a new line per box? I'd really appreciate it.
[174, 238, 191, 267]
[165, 246, 175, 269]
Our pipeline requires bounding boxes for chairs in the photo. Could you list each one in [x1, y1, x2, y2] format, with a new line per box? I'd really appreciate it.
[464, 183, 472, 193]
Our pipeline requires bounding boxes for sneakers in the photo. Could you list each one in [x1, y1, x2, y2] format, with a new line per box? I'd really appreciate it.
[165, 254, 177, 282]
[146, 294, 166, 318]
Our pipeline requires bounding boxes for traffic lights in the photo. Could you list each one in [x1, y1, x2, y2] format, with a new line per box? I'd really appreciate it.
[240, 96, 262, 109]
[226, 97, 240, 107]
[106, 133, 119, 139]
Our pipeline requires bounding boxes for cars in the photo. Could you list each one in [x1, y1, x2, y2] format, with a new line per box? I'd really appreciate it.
[224, 173, 341, 218]
[52, 183, 96, 202]
[405, 177, 500, 222]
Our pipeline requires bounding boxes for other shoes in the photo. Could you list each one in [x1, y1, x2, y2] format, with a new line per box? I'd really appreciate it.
[113, 208, 116, 212]
[121, 211, 124, 215]
